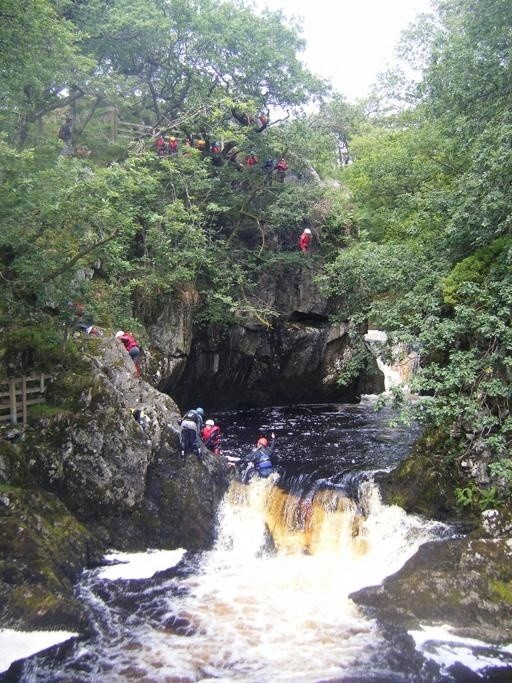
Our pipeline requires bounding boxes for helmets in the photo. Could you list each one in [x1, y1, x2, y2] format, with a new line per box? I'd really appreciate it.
[197, 407, 204, 415]
[259, 438, 266, 446]
[205, 418, 215, 426]
[115, 331, 124, 338]
[304, 228, 311, 233]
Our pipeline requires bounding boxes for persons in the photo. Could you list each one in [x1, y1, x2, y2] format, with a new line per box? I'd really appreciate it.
[155, 127, 287, 187]
[60, 116, 73, 161]
[243, 112, 249, 125]
[178, 406, 205, 462]
[299, 228, 313, 256]
[202, 420, 224, 457]
[228, 432, 277, 479]
[259, 112, 267, 125]
[117, 330, 140, 366]
